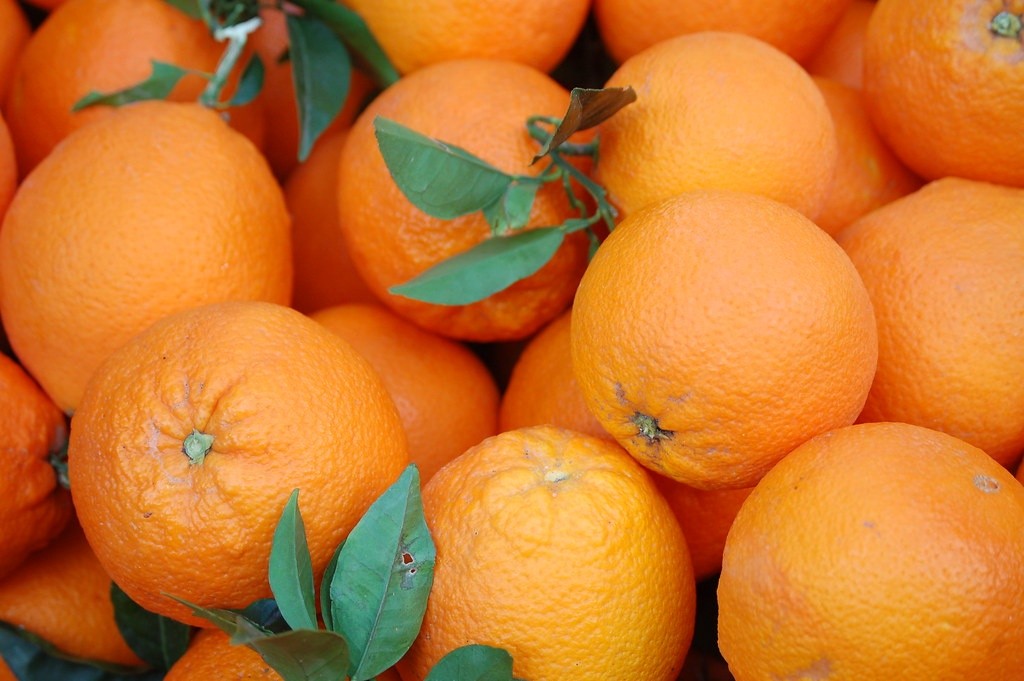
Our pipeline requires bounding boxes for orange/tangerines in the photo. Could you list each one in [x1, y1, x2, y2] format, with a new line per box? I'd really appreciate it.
[0, 0, 1024, 681]
